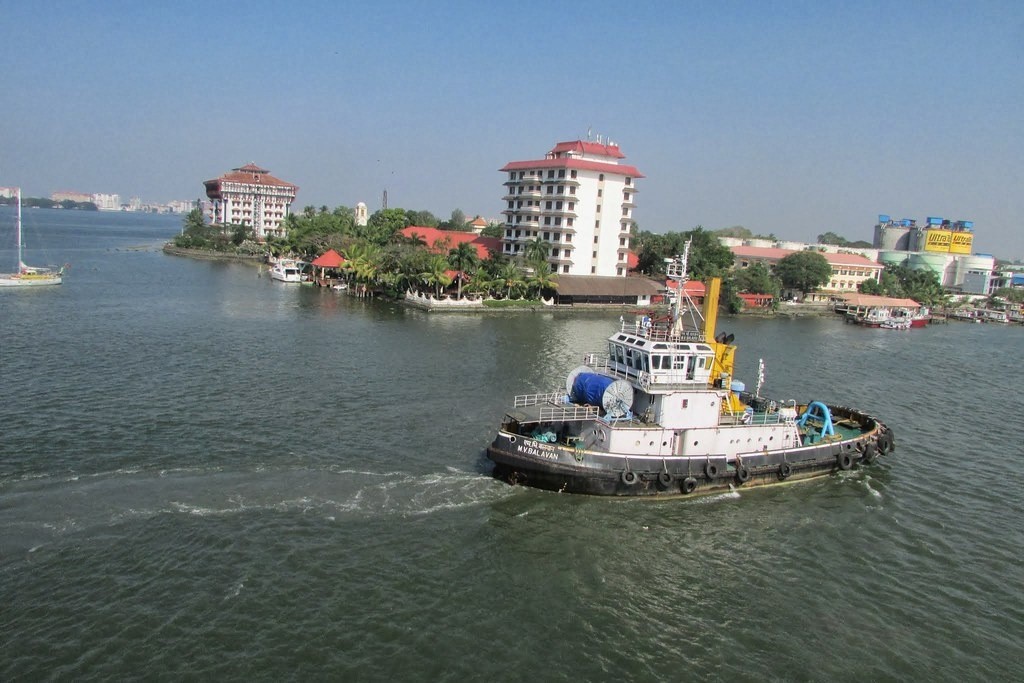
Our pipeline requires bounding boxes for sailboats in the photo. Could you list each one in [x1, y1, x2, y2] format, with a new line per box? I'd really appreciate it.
[0, 187, 63, 286]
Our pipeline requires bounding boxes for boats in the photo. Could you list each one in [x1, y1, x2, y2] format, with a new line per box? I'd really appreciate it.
[485, 241, 896, 495]
[845, 307, 933, 329]
[268, 258, 300, 283]
